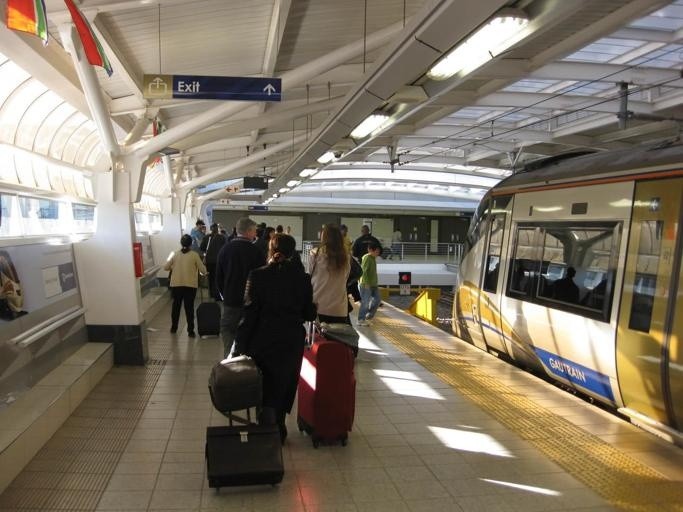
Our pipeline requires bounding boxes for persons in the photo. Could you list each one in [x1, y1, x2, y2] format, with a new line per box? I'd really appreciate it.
[227, 232, 318, 447]
[388, 229, 404, 261]
[162, 217, 385, 361]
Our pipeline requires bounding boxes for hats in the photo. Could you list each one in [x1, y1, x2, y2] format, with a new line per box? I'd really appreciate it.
[196, 220, 204, 225]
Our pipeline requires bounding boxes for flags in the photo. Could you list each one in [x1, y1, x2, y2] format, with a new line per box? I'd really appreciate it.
[7, 1, 49, 48]
[65, 0, 113, 77]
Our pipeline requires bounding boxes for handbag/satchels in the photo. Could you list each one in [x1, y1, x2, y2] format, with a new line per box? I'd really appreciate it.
[315, 321, 359, 354]
[208, 351, 264, 411]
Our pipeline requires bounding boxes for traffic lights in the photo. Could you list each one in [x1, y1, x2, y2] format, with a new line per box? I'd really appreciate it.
[398, 271, 411, 285]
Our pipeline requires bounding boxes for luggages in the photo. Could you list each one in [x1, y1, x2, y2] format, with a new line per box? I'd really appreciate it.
[297, 319, 356, 449]
[197, 277, 220, 337]
[205, 409, 285, 490]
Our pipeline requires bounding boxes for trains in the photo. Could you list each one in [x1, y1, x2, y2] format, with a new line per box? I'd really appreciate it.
[448, 135, 682, 444]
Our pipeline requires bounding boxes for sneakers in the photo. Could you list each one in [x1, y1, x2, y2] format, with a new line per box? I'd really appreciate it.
[357, 320, 369, 326]
